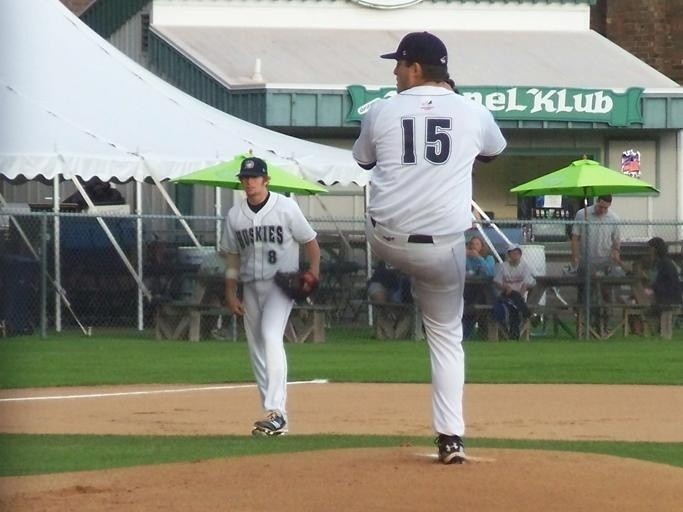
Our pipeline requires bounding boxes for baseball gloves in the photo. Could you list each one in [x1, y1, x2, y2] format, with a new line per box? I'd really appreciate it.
[274, 269, 319, 301]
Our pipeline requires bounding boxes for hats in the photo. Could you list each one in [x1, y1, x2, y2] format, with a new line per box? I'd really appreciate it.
[235, 157, 268, 179]
[378, 31, 448, 68]
[506, 243, 522, 252]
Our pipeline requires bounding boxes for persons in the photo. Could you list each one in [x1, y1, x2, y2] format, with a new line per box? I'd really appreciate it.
[621, 236, 682, 338]
[568, 193, 623, 339]
[348, 27, 509, 465]
[217, 154, 323, 439]
[365, 235, 541, 341]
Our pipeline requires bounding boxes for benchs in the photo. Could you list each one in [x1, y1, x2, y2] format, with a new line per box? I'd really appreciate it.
[181, 273, 643, 343]
[150, 302, 683, 343]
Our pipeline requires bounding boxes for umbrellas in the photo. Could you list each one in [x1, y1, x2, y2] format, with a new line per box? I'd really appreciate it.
[506, 152, 661, 339]
[167, 155, 329, 197]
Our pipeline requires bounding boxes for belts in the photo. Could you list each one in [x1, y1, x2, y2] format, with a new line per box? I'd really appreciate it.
[370, 218, 433, 244]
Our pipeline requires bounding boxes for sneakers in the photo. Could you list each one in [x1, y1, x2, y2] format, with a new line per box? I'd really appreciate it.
[362, 265, 400, 298]
[530, 312, 545, 328]
[434, 434, 468, 463]
[250, 413, 288, 437]
